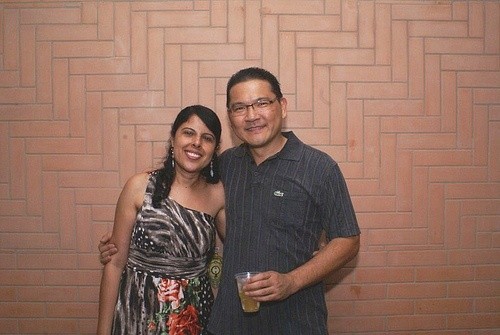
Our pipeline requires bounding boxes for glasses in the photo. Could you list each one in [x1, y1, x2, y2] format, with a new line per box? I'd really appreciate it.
[227, 97, 279, 116]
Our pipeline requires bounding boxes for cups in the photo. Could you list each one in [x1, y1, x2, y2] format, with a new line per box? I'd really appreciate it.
[233, 272, 261, 313]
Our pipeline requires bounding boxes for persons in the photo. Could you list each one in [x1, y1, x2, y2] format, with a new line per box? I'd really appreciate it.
[98, 67, 361, 335]
[96, 105, 320, 335]
